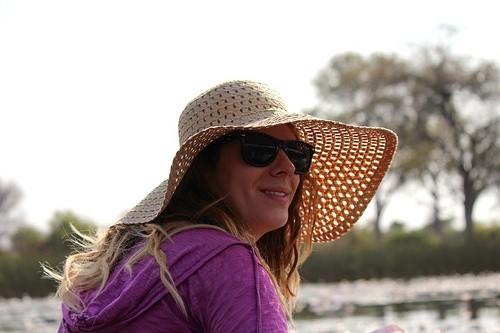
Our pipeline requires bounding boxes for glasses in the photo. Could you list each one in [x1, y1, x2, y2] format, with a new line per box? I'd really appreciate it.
[233, 131, 316, 176]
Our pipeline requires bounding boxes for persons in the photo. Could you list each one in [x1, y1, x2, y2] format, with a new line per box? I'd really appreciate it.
[56, 78, 331, 332]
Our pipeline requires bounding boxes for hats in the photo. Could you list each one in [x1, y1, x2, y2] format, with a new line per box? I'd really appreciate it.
[108, 80, 395, 242]
